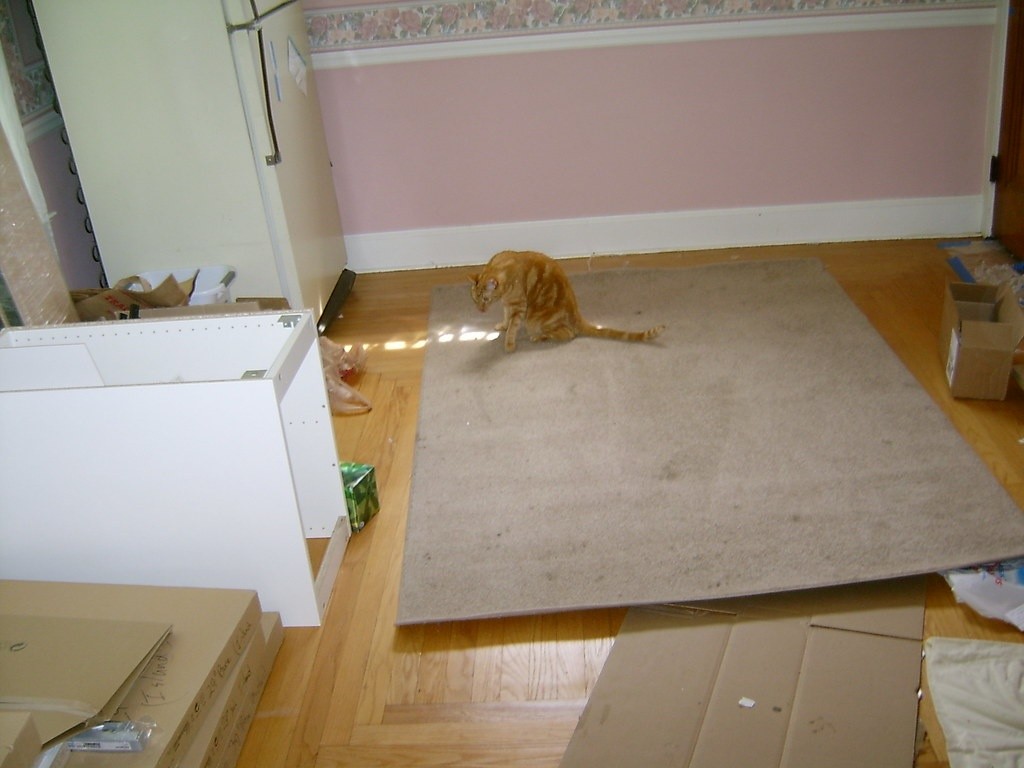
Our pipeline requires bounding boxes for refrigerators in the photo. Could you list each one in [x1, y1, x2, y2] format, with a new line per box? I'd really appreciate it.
[32, 0, 355, 337]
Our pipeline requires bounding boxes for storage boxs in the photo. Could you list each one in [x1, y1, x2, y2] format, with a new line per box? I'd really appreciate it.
[335, 462, 381, 534]
[0, 579, 285, 768]
[936, 241, 1024, 398]
[938, 280, 1024, 402]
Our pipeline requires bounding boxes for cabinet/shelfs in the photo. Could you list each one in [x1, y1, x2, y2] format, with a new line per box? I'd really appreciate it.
[1, 311, 354, 626]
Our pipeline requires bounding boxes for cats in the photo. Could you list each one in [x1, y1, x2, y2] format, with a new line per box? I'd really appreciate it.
[468, 249, 667, 355]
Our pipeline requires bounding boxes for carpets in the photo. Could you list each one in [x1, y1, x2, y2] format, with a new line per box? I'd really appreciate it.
[393, 260, 1024, 626]
